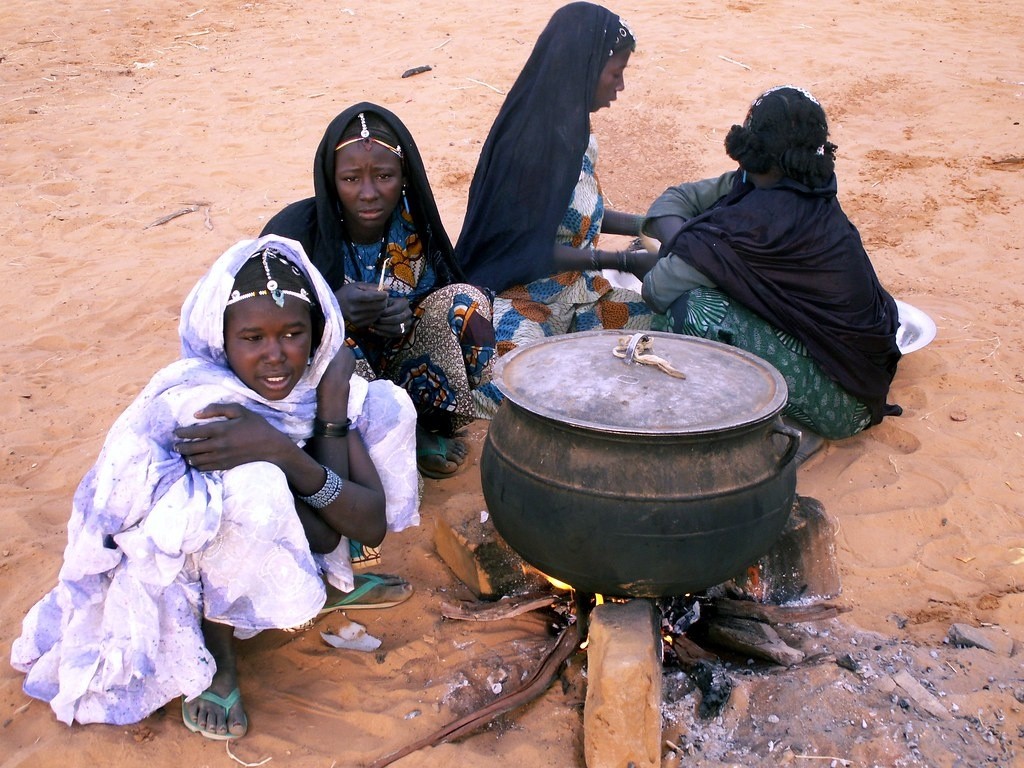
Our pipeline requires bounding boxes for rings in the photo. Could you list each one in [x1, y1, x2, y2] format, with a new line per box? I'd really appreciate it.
[400, 323, 405, 333]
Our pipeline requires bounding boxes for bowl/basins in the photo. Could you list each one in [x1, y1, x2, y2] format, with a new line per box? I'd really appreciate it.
[892, 298, 939, 356]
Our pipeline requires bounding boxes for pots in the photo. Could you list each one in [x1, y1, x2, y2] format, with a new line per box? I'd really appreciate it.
[480, 330, 805, 601]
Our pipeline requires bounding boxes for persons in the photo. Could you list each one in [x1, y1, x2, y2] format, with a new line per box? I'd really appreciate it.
[453, 1, 661, 418]
[641, 87, 904, 464]
[257, 101, 501, 479]
[12, 235, 421, 741]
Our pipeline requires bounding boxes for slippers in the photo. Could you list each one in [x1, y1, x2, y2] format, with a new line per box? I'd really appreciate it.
[180, 689, 248, 741]
[320, 572, 415, 613]
[415, 434, 462, 479]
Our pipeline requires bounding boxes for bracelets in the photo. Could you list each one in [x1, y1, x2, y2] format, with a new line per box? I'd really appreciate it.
[589, 249, 603, 271]
[313, 415, 351, 437]
[296, 464, 343, 508]
[616, 249, 638, 273]
